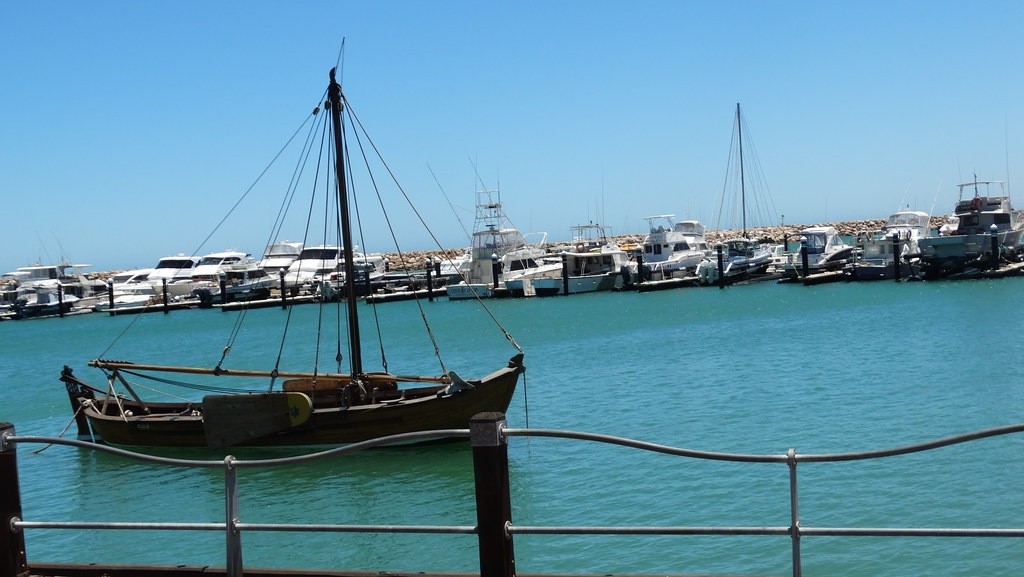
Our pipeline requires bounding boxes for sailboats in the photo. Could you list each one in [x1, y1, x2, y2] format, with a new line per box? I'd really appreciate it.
[59, 67, 526, 450]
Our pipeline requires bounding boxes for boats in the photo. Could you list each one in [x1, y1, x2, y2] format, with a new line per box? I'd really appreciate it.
[0, 100, 1024, 321]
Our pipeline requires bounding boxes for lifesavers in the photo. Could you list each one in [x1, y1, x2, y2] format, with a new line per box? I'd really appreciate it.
[577, 242, 584, 253]
[906, 230, 912, 240]
